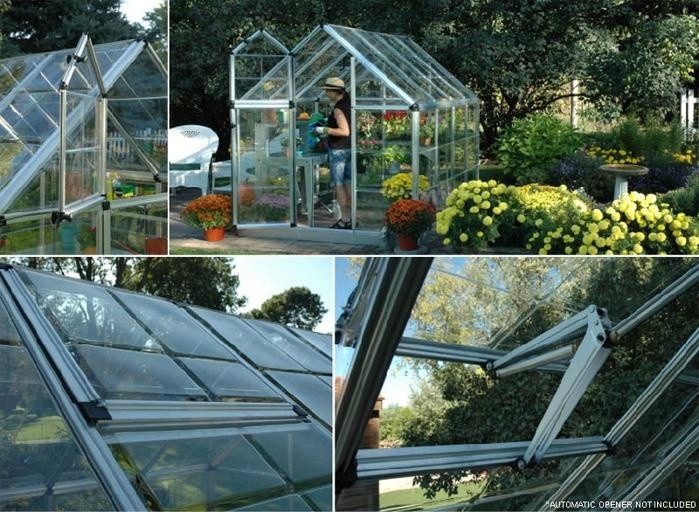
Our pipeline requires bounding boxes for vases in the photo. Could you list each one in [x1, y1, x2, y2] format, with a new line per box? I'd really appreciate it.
[400, 230, 419, 250]
[202, 226, 225, 242]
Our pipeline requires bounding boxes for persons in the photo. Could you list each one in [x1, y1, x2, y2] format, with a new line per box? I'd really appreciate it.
[312, 75, 355, 232]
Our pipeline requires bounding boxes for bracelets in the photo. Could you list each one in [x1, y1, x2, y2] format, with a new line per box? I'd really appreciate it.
[316, 126, 330, 134]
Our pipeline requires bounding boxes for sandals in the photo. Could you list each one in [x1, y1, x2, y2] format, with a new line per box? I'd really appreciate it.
[329, 218, 359, 229]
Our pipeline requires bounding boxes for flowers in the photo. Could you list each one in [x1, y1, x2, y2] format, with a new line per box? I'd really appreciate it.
[386, 199, 438, 235]
[179, 192, 234, 230]
[379, 170, 429, 199]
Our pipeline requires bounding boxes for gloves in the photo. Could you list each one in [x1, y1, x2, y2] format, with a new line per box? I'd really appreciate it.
[311, 125, 325, 136]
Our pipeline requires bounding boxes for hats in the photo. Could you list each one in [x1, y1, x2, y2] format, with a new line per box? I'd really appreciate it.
[321, 77, 345, 89]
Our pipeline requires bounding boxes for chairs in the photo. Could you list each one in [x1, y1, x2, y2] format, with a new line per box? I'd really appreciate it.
[168, 124, 220, 199]
